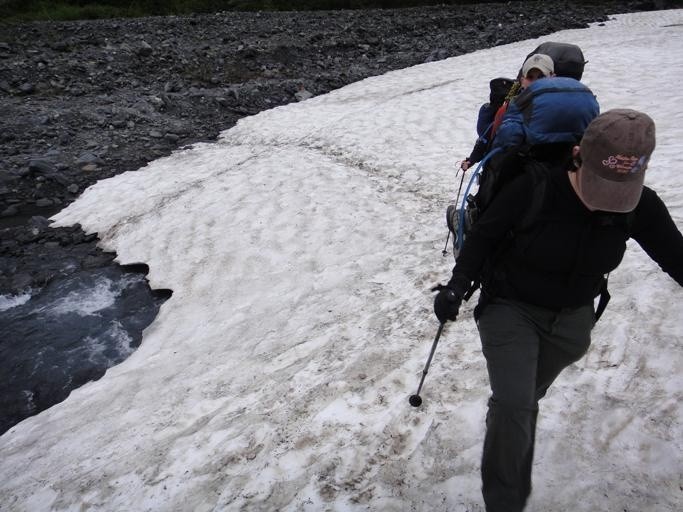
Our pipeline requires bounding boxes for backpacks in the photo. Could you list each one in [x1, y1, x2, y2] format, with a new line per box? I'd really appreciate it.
[475, 77, 600, 211]
[474, 42, 585, 137]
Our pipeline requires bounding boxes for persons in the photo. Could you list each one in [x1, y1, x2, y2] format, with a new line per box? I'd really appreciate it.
[432, 109, 683, 511]
[459, 53, 557, 172]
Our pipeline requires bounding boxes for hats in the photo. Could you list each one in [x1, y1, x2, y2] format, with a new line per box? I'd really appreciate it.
[523, 53, 555, 77]
[581, 108, 656, 213]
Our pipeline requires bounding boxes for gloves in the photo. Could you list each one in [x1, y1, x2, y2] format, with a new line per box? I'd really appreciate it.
[434, 274, 467, 322]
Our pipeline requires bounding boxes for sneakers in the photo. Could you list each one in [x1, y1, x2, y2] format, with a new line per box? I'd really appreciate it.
[447, 205, 471, 262]
